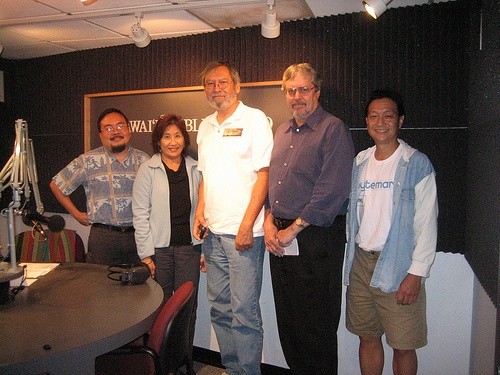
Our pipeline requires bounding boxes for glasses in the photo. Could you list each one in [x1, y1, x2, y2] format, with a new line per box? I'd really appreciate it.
[101, 123, 128, 132]
[204, 78, 233, 89]
[285, 86, 315, 97]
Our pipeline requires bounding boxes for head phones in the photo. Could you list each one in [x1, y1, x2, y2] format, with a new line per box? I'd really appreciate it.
[108, 260, 154, 282]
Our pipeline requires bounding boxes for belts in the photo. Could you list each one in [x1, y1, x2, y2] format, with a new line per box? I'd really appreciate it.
[91, 222, 136, 234]
[273, 216, 295, 229]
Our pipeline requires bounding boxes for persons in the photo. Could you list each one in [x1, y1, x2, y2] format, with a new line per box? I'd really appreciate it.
[344, 92, 438, 375]
[193, 60, 274, 375]
[263, 63, 355, 375]
[133, 114, 202, 375]
[50, 109, 151, 268]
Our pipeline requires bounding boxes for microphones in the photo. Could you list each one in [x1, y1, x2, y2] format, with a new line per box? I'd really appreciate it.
[15, 209, 65, 232]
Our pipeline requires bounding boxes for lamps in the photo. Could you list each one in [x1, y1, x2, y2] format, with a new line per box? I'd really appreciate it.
[131, 12, 151, 49]
[362, 0, 393, 19]
[261, 1, 279, 39]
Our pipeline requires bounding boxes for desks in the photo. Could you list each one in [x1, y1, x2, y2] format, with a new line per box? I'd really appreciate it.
[0, 262, 165, 375]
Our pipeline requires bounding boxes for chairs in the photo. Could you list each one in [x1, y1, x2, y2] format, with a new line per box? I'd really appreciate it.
[5, 229, 86, 262]
[95, 281, 196, 375]
